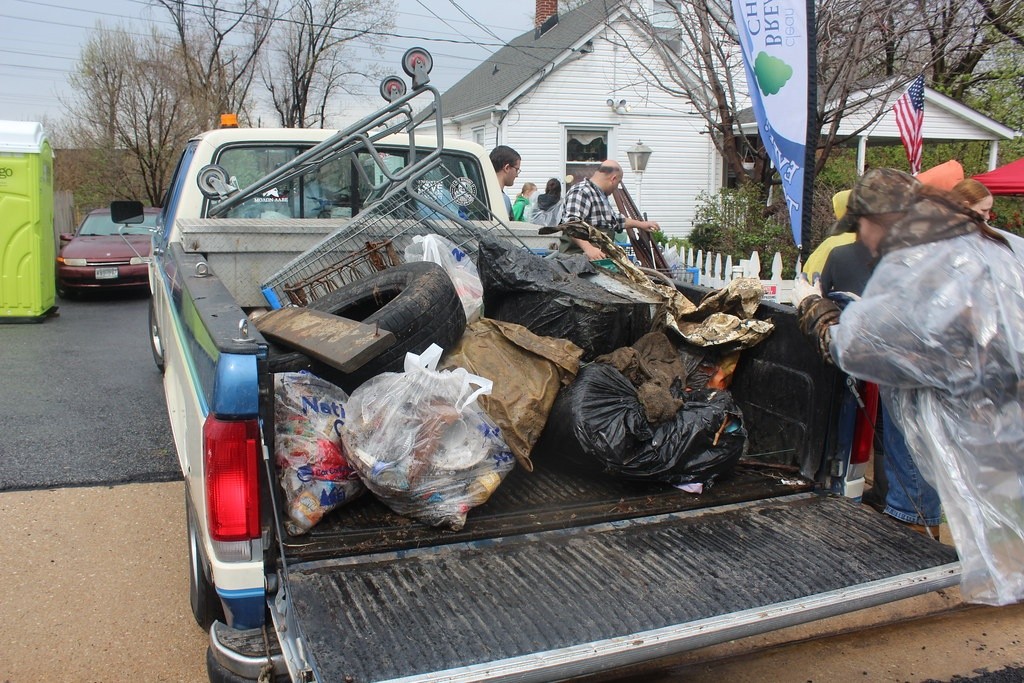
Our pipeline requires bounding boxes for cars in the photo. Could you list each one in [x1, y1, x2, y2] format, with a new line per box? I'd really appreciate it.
[54, 207, 166, 298]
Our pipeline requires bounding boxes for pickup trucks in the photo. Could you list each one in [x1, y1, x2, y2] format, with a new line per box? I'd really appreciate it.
[108, 113, 996, 682]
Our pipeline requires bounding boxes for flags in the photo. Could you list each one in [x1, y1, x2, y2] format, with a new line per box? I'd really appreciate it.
[890, 69, 925, 176]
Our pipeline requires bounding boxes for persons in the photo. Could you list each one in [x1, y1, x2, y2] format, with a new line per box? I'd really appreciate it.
[457, 144, 660, 262]
[788, 167, 1024, 542]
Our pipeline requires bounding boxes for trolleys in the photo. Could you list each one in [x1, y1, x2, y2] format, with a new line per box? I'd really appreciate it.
[195, 46, 546, 310]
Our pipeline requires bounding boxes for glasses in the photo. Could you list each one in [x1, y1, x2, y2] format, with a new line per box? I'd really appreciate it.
[507, 164, 521, 174]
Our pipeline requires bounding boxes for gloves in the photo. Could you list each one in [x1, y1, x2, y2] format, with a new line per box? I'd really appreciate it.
[789, 271, 823, 309]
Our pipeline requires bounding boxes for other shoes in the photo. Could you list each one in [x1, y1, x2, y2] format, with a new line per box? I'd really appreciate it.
[885, 515, 940, 541]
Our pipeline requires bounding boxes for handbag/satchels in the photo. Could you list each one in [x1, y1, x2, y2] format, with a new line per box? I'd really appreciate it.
[270, 231, 748, 535]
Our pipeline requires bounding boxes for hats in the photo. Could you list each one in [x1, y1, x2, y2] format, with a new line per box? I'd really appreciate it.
[830, 166, 922, 235]
